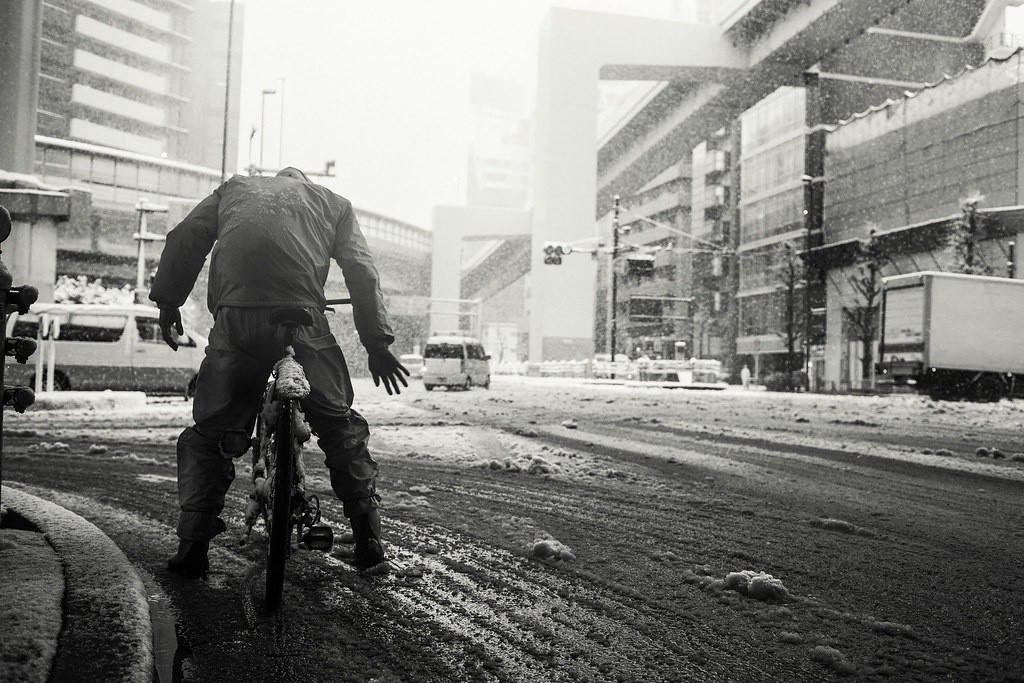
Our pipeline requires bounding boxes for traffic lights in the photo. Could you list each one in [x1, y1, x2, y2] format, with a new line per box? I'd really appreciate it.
[544, 245, 572, 265]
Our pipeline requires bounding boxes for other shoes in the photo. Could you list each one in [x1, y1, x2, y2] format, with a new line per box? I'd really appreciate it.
[168, 555, 209, 581]
[355, 537, 384, 569]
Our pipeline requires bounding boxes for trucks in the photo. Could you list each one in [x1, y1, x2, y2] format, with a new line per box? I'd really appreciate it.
[874, 271, 1024, 402]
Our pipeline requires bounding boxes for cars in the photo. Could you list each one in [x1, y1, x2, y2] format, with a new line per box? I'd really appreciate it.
[762, 369, 810, 392]
[400, 354, 423, 379]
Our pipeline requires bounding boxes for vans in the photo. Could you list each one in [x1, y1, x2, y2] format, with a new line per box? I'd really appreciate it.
[422, 336, 491, 391]
[3, 304, 209, 401]
[592, 353, 631, 379]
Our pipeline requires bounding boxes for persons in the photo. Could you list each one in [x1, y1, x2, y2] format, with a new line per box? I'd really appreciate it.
[148, 166, 409, 578]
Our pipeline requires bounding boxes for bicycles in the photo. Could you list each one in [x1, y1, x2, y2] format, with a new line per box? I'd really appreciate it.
[248, 299, 354, 609]
